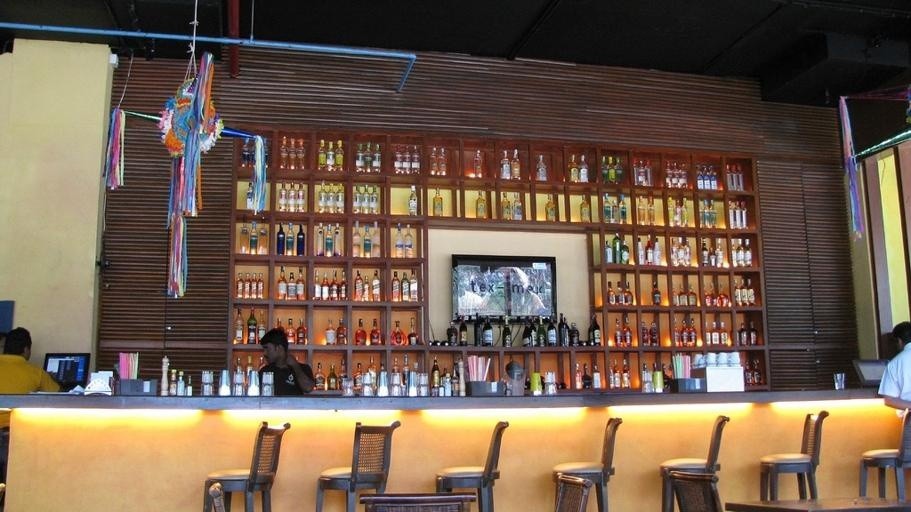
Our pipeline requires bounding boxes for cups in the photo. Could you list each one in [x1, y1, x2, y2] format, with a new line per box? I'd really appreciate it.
[508, 368, 527, 397]
[693, 352, 741, 364]
[529, 370, 557, 394]
[833, 373, 846, 390]
[200, 367, 274, 397]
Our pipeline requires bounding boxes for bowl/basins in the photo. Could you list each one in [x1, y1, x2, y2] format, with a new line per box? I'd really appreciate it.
[470, 381, 506, 398]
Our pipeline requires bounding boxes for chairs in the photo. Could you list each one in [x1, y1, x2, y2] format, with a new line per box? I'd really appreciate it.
[669, 471, 726, 512]
[316, 419, 401, 512]
[359, 492, 476, 512]
[554, 474, 592, 512]
[203, 421, 291, 511]
[760, 411, 830, 500]
[661, 415, 730, 512]
[436, 421, 509, 512]
[553, 417, 623, 512]
[860, 408, 911, 501]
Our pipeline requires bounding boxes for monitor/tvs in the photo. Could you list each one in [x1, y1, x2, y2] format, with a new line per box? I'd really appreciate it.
[451, 254, 559, 324]
[849, 358, 892, 387]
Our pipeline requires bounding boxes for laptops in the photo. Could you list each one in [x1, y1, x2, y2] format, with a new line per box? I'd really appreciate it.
[42, 352, 90, 393]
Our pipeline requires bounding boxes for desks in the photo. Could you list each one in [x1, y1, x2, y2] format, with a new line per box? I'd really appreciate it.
[724, 496, 911, 512]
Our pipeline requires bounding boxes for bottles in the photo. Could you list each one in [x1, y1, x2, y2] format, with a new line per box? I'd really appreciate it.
[161, 355, 193, 397]
[574, 356, 671, 394]
[236, 214, 421, 348]
[445, 315, 601, 348]
[743, 359, 763, 386]
[239, 135, 750, 229]
[232, 354, 467, 398]
[603, 232, 760, 350]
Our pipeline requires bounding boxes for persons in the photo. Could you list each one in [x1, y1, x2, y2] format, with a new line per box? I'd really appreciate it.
[0, 328, 64, 484]
[256, 327, 316, 395]
[878, 321, 911, 469]
[458, 265, 551, 313]
